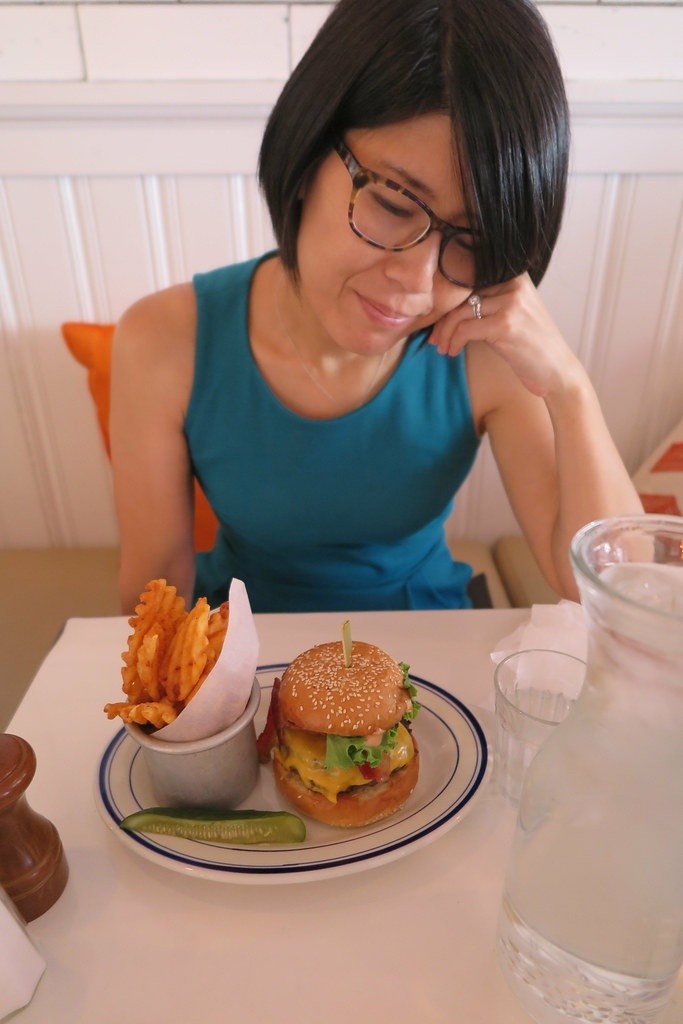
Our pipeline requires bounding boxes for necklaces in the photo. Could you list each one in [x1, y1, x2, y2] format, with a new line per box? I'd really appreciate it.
[273, 291, 388, 412]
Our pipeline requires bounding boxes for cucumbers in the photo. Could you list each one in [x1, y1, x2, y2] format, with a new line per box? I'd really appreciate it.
[119, 806, 305, 844]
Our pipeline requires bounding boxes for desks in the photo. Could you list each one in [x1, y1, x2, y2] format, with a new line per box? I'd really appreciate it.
[0, 605, 683, 1024]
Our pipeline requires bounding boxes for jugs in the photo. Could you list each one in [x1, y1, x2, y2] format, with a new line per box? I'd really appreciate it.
[495, 513, 683, 1023]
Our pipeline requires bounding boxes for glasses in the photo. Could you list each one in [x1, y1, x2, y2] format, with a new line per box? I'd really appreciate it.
[331, 142, 507, 290]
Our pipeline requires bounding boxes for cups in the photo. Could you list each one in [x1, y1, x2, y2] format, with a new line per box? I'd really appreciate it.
[490, 648, 589, 806]
[124, 674, 262, 812]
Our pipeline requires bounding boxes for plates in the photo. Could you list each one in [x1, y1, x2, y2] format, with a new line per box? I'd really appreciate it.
[92, 661, 493, 885]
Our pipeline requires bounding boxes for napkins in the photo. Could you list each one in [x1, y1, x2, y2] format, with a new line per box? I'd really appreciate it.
[152, 578, 259, 742]
[490, 599, 592, 697]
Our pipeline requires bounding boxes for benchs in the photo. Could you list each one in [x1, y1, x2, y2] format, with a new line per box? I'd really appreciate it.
[447, 535, 564, 609]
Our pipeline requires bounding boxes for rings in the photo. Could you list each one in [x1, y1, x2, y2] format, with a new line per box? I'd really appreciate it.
[465, 293, 484, 320]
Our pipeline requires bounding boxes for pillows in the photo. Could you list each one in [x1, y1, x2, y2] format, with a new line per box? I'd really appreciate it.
[63, 320, 219, 548]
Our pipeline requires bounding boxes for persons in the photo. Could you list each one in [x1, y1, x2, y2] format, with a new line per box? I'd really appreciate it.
[108, 0, 656, 618]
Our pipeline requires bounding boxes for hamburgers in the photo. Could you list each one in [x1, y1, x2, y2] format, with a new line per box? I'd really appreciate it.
[272, 641, 421, 827]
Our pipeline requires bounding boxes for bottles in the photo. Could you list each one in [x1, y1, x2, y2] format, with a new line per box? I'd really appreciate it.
[0, 882, 47, 1023]
